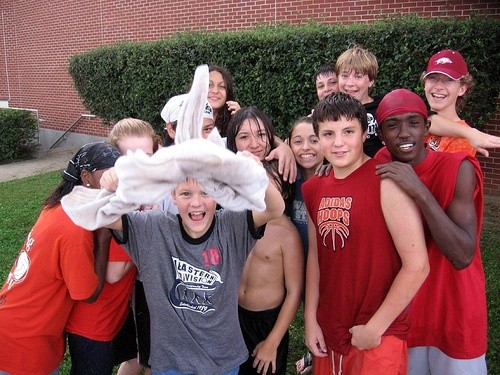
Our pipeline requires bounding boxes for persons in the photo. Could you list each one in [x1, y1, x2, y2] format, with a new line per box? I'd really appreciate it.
[314, 88, 487, 375]
[99, 167, 285, 375]
[300, 91, 430, 375]
[0, 143, 121, 375]
[65, 50, 500, 375]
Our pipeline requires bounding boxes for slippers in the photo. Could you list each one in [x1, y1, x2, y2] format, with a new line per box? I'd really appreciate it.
[295, 351, 314, 375]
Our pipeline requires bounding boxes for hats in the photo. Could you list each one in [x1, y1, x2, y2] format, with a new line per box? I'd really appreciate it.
[422, 49, 468, 81]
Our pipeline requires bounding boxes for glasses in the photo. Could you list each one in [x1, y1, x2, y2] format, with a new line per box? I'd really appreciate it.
[426, 75, 461, 86]
